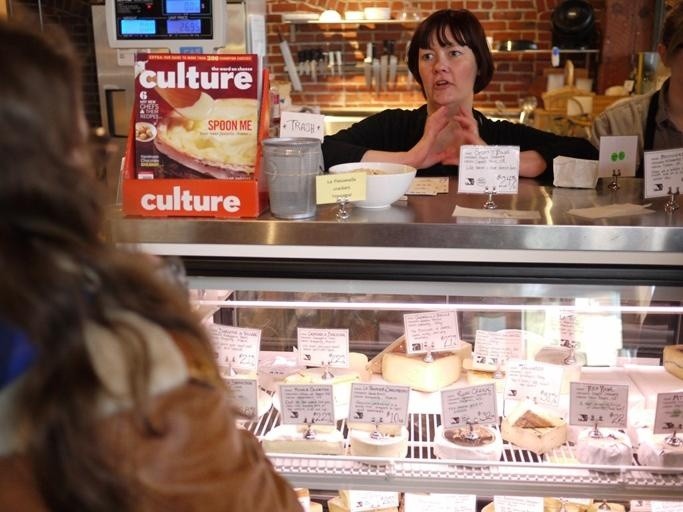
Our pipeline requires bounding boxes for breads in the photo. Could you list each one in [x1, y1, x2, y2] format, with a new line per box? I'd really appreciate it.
[156, 99, 255, 179]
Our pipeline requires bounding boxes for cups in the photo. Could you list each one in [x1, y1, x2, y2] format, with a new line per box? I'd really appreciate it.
[261, 137, 321, 220]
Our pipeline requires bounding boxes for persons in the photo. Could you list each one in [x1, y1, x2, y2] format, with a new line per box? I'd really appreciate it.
[591, 5, 683, 175]
[0, 14, 299, 512]
[321, 9, 598, 188]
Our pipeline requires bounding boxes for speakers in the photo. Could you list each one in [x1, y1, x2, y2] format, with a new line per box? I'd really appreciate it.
[551, 0, 595, 49]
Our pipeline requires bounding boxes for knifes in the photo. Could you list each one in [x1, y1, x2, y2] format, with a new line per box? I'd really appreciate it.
[275, 24, 412, 96]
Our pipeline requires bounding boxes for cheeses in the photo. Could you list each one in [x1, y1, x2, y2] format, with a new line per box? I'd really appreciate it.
[223, 341, 683, 512]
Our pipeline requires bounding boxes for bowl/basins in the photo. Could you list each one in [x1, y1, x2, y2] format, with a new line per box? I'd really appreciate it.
[320, 7, 392, 21]
[327, 162, 417, 211]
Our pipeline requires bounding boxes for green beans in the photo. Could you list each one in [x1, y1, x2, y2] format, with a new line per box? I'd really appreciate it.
[136, 126, 153, 139]
